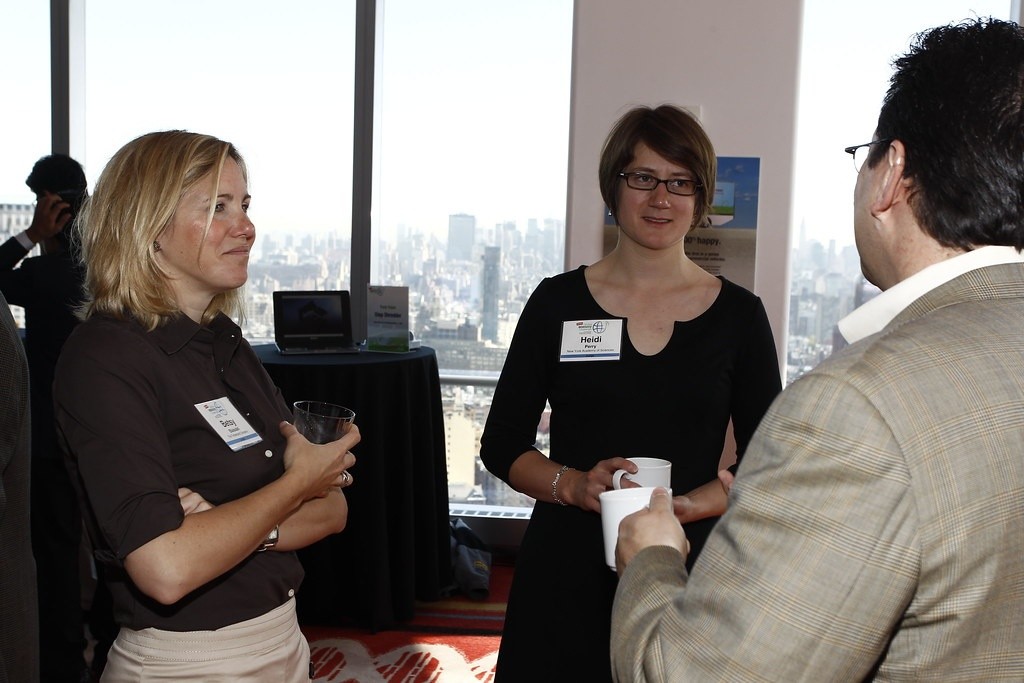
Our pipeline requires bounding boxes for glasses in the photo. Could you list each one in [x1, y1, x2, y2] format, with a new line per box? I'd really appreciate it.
[618, 172, 700, 196]
[844, 136, 892, 172]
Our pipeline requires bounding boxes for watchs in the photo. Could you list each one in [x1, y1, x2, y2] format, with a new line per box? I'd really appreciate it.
[256, 524, 279, 553]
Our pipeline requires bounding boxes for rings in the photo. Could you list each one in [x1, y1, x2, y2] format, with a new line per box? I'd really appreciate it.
[340, 471, 349, 489]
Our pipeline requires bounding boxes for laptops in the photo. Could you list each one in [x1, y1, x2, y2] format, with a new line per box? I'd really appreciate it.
[273, 290, 360, 355]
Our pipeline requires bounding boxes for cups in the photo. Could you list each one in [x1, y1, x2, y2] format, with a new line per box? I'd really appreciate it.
[613, 458, 671, 490]
[293, 400, 356, 446]
[599, 487, 674, 572]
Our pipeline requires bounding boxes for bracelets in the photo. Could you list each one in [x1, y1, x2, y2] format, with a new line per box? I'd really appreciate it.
[551, 464, 576, 507]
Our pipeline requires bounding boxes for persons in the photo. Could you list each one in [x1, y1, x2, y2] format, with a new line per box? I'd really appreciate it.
[0, 130, 361, 683]
[480, 104, 783, 683]
[610, 17, 1024, 683]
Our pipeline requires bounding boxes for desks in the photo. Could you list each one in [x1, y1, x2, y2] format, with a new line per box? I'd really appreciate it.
[249, 342, 454, 628]
